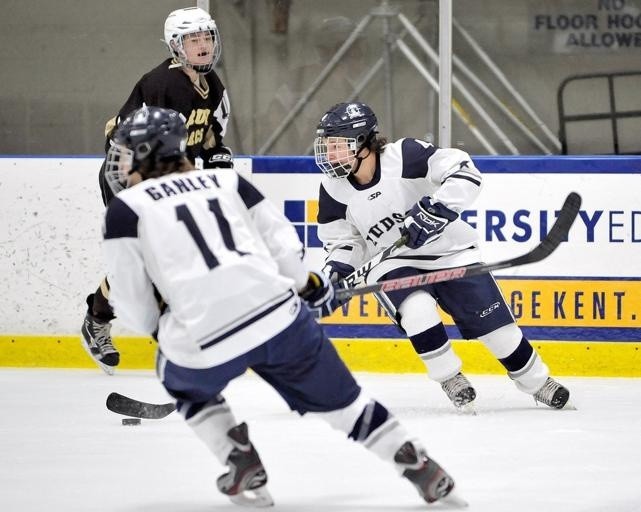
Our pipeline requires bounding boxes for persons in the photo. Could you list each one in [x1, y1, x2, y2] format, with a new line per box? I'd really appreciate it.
[316, 103, 577, 410]
[82, 7, 230, 376]
[101, 106, 468, 510]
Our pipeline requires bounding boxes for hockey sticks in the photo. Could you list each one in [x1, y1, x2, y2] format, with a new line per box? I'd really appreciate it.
[107, 234, 411, 419]
[336, 192, 581, 300]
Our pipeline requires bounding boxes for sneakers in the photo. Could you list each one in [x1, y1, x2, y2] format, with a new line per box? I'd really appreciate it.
[82, 315, 120, 367]
[532, 377, 568, 409]
[441, 372, 475, 405]
[402, 452, 454, 504]
[217, 441, 268, 495]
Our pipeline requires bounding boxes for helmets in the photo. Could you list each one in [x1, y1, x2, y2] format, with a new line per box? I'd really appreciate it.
[164, 7, 222, 74]
[314, 102, 377, 179]
[103, 105, 188, 196]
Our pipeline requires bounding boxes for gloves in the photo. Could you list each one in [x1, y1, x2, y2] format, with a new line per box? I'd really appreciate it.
[399, 195, 460, 249]
[299, 261, 355, 318]
